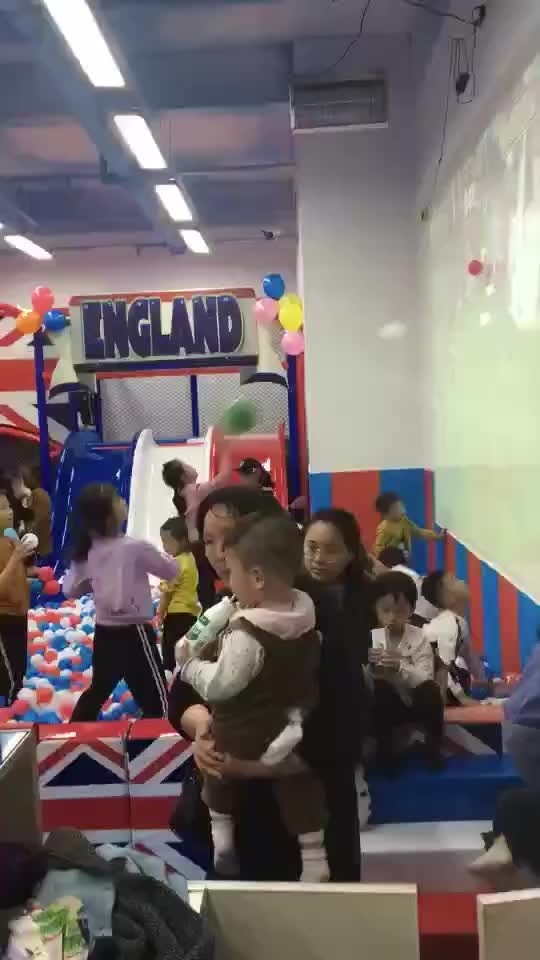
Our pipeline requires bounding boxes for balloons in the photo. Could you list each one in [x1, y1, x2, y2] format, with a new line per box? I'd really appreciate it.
[468, 260, 483, 275]
[254, 298, 278, 322]
[222, 400, 258, 434]
[278, 292, 304, 332]
[281, 331, 304, 356]
[44, 311, 67, 331]
[15, 311, 41, 334]
[31, 286, 54, 313]
[263, 273, 286, 299]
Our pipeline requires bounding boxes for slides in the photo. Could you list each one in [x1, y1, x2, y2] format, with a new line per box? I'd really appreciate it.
[47, 419, 291, 594]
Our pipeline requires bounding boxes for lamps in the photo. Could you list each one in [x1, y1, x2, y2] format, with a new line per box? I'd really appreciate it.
[0, 0, 216, 261]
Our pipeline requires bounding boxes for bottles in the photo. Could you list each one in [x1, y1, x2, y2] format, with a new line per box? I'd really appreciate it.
[175, 595, 236, 657]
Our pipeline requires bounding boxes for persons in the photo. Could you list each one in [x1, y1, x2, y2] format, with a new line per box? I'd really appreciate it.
[164, 443, 239, 608]
[159, 516, 202, 673]
[378, 547, 483, 708]
[366, 572, 451, 777]
[467, 635, 540, 876]
[63, 482, 179, 724]
[0, 461, 51, 708]
[297, 509, 376, 885]
[233, 457, 271, 495]
[373, 494, 445, 562]
[170, 485, 357, 882]
